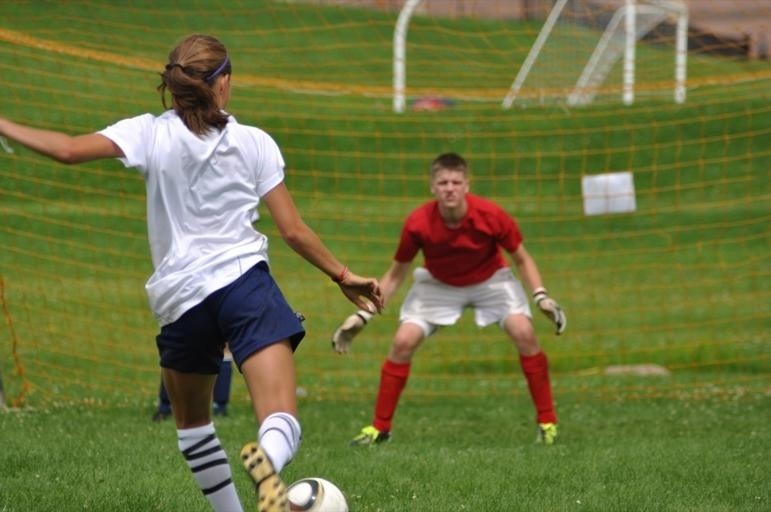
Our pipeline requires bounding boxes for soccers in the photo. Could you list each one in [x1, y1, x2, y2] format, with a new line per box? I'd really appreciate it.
[287, 477, 348, 511]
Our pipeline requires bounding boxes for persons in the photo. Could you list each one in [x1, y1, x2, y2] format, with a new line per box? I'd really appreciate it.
[332, 153, 567, 447]
[152, 342, 233, 424]
[0, 35, 384, 512]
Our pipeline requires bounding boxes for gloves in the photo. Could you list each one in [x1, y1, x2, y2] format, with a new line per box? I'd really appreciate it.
[532, 287, 567, 336]
[331, 308, 372, 355]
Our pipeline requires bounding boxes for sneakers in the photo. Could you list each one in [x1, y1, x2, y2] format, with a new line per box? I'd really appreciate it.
[537, 422, 558, 445]
[240, 442, 290, 512]
[348, 424, 390, 446]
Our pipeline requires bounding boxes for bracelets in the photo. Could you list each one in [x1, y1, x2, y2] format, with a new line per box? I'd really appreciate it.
[332, 266, 348, 282]
